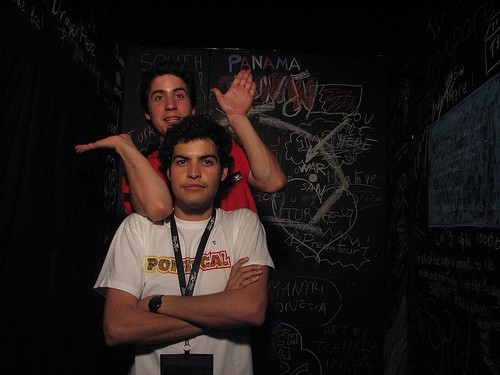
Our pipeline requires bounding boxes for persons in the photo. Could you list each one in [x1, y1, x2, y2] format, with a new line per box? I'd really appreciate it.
[94, 114, 275, 375]
[75, 61, 286, 221]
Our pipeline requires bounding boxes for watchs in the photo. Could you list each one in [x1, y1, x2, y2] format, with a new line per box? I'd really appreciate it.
[148, 295, 165, 313]
